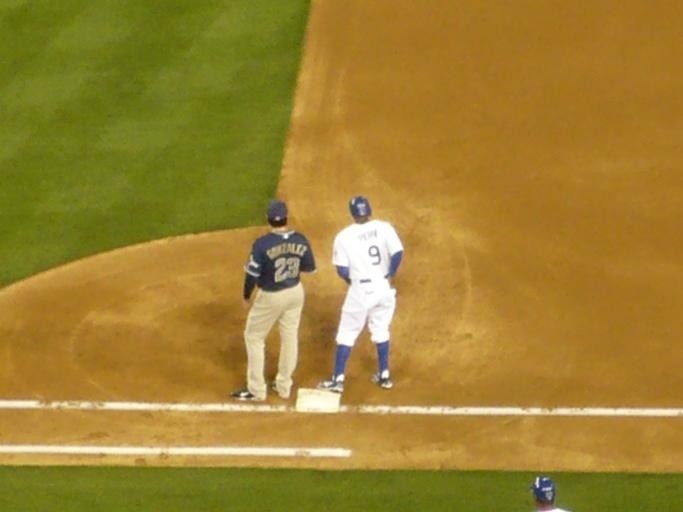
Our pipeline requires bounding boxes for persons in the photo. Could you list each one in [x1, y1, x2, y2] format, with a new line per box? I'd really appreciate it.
[315, 195, 404, 392]
[228, 200, 318, 400]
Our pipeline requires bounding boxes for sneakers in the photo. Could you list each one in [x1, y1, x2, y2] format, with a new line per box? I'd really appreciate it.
[316, 378, 344, 391]
[371, 373, 393, 388]
[273, 380, 289, 397]
[231, 386, 267, 400]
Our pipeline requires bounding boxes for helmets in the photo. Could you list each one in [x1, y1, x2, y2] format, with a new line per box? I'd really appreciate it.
[266, 200, 287, 222]
[349, 196, 370, 218]
[533, 472, 555, 499]
